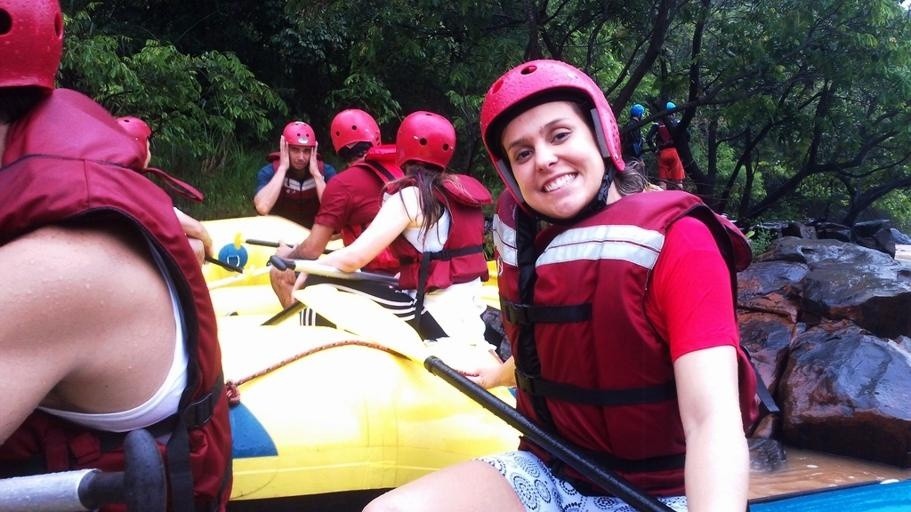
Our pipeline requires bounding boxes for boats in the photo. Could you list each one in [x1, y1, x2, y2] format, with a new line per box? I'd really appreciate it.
[750, 475, 911, 510]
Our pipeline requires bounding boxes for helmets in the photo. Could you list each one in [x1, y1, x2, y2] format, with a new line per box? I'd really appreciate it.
[629, 104, 646, 121]
[115, 113, 152, 169]
[394, 109, 458, 170]
[666, 101, 677, 111]
[281, 120, 318, 148]
[327, 107, 383, 159]
[0, 0, 66, 96]
[478, 58, 628, 222]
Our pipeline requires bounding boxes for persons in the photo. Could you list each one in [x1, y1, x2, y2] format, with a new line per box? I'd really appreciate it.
[622, 103, 645, 176]
[358, 58, 776, 512]
[0, 0, 233, 512]
[647, 102, 683, 190]
[254, 121, 336, 228]
[269, 109, 404, 310]
[290, 113, 494, 307]
[110, 116, 153, 178]
